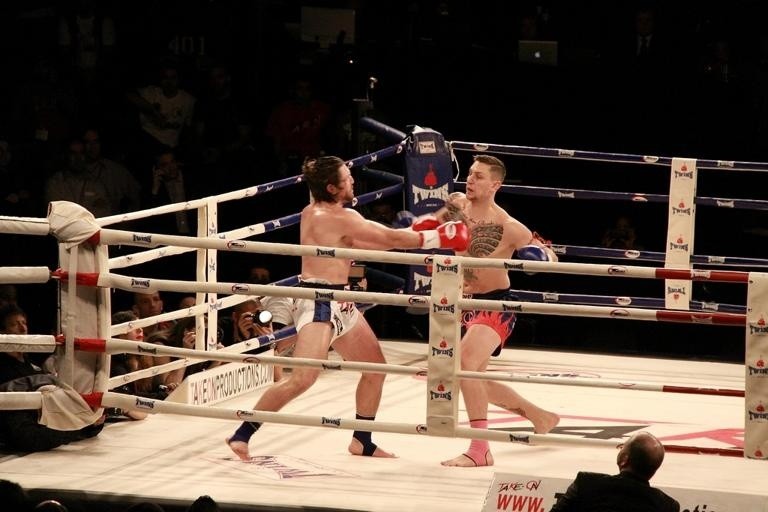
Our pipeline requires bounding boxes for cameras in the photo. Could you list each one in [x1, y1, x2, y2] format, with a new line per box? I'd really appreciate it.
[195, 328, 224, 345]
[243, 310, 273, 331]
[348, 265, 366, 305]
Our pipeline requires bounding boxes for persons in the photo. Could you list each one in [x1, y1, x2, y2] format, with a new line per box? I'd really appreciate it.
[185, 495, 221, 512]
[415, 154, 560, 468]
[549, 430, 681, 512]
[0, 478, 32, 512]
[34, 499, 68, 512]
[126, 501, 164, 512]
[1, 65, 337, 401]
[603, 217, 637, 249]
[224, 154, 472, 464]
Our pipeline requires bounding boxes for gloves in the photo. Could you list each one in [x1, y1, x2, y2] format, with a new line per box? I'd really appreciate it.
[517, 245, 555, 277]
[409, 213, 472, 252]
